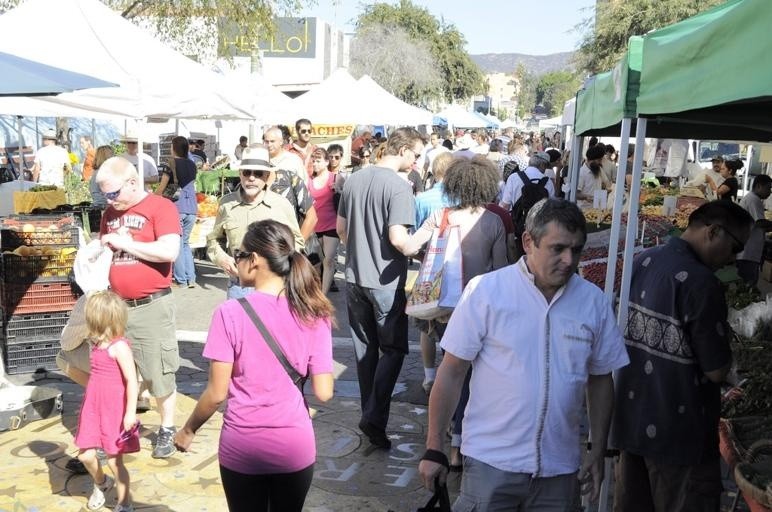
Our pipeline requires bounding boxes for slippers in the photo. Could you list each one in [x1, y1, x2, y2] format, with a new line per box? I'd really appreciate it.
[447, 425, 463, 473]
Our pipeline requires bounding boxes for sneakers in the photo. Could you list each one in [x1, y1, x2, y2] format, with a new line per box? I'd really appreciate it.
[86, 475, 114, 511]
[151, 425, 176, 459]
[358, 419, 391, 449]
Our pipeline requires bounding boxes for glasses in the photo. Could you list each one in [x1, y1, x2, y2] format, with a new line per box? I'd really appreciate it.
[300, 129, 311, 134]
[241, 170, 265, 178]
[234, 249, 251, 264]
[100, 182, 125, 200]
[330, 155, 340, 161]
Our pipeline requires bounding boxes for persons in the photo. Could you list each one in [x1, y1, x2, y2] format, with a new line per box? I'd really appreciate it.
[33, 118, 772, 512]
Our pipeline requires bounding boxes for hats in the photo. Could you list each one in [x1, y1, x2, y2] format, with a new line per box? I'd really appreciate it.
[41, 130, 57, 140]
[236, 146, 276, 174]
[119, 130, 138, 144]
[711, 155, 724, 162]
[532, 150, 552, 170]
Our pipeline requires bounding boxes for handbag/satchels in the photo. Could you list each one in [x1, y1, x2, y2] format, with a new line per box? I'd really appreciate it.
[297, 214, 325, 266]
[160, 183, 183, 201]
[333, 192, 341, 214]
[405, 224, 463, 321]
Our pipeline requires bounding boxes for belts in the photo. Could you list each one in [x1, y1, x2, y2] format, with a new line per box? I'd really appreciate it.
[125, 287, 172, 308]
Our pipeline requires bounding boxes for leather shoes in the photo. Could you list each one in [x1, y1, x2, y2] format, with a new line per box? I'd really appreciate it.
[422, 376, 435, 396]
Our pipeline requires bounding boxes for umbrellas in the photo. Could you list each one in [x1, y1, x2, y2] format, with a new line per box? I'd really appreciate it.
[0, 51, 120, 97]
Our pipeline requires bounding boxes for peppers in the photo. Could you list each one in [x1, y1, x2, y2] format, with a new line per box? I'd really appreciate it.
[580, 239, 641, 292]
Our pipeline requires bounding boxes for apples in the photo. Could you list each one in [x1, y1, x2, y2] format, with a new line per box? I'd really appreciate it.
[3, 245, 75, 282]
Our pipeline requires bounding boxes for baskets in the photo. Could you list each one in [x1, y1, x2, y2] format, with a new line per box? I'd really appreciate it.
[734, 440, 771, 511]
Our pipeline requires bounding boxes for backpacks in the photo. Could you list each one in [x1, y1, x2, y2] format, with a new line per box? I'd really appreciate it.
[512, 171, 549, 246]
